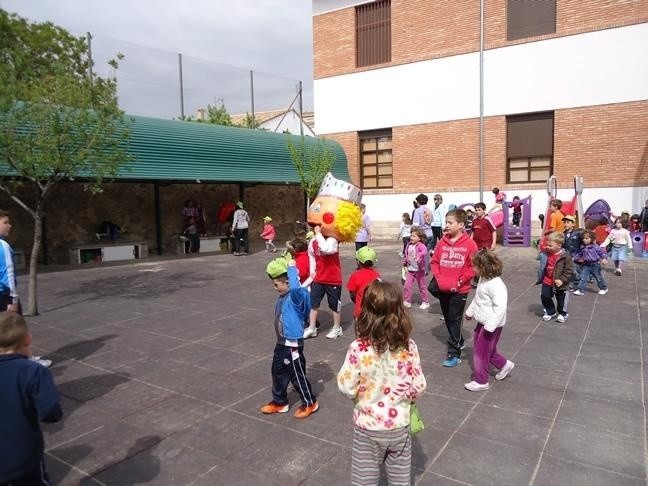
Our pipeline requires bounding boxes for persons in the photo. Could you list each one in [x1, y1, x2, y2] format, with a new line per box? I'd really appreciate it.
[430, 208, 478, 368]
[463, 247, 515, 393]
[545, 200, 648, 296]
[509, 196, 522, 227]
[304, 172, 364, 340]
[336, 279, 427, 485]
[94, 220, 128, 242]
[347, 245, 382, 335]
[262, 252, 319, 418]
[493, 188, 506, 206]
[355, 204, 371, 250]
[0, 210, 52, 369]
[181, 199, 250, 257]
[402, 226, 430, 309]
[536, 231, 574, 323]
[259, 216, 278, 252]
[0, 310, 63, 486]
[397, 192, 496, 287]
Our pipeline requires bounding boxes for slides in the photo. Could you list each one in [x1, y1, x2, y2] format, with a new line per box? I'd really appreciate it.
[537, 195, 577, 252]
[489, 211, 503, 228]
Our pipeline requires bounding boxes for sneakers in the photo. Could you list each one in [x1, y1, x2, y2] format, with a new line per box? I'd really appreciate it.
[398, 253, 404, 259]
[261, 403, 290, 413]
[495, 360, 515, 381]
[420, 302, 429, 310]
[542, 259, 622, 323]
[303, 327, 319, 339]
[30, 356, 53, 369]
[403, 301, 411, 309]
[464, 381, 489, 392]
[294, 401, 319, 419]
[439, 352, 462, 367]
[326, 326, 343, 338]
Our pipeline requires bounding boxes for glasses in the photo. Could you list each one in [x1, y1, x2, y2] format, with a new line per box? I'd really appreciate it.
[433, 197, 437, 200]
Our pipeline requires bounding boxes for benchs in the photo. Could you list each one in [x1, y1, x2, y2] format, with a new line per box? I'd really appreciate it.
[67, 241, 152, 265]
[168, 234, 236, 256]
[11, 248, 26, 272]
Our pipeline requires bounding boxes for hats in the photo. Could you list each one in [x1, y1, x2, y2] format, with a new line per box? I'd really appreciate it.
[560, 215, 576, 223]
[356, 246, 377, 265]
[262, 216, 272, 222]
[263, 252, 293, 278]
[236, 201, 244, 210]
[409, 405, 425, 439]
